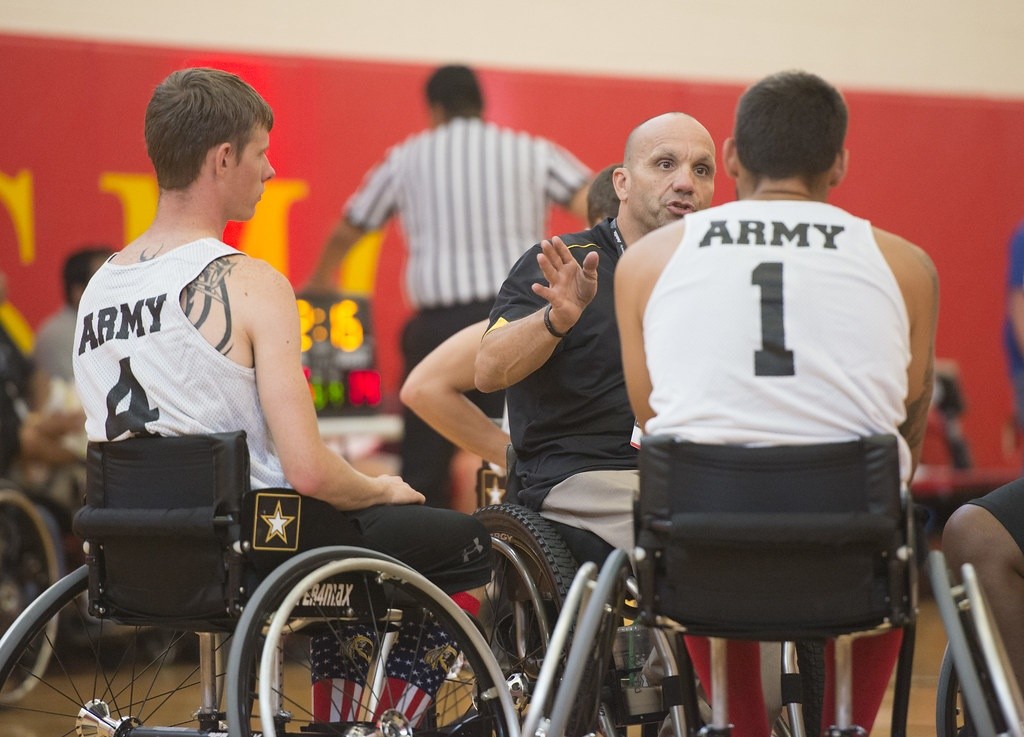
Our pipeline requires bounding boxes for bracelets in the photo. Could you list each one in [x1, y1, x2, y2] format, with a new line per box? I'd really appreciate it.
[545, 304, 576, 338]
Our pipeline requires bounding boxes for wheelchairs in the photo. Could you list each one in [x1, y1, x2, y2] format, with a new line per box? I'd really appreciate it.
[0, 415, 700, 737]
[527, 439, 1024, 736]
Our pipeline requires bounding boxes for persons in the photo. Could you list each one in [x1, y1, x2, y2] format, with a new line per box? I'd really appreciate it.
[310, 64, 598, 513]
[73, 66, 493, 728]
[398, 163, 622, 469]
[613, 71, 937, 737]
[475, 113, 717, 568]
[0, 248, 113, 627]
[940, 476, 1024, 699]
